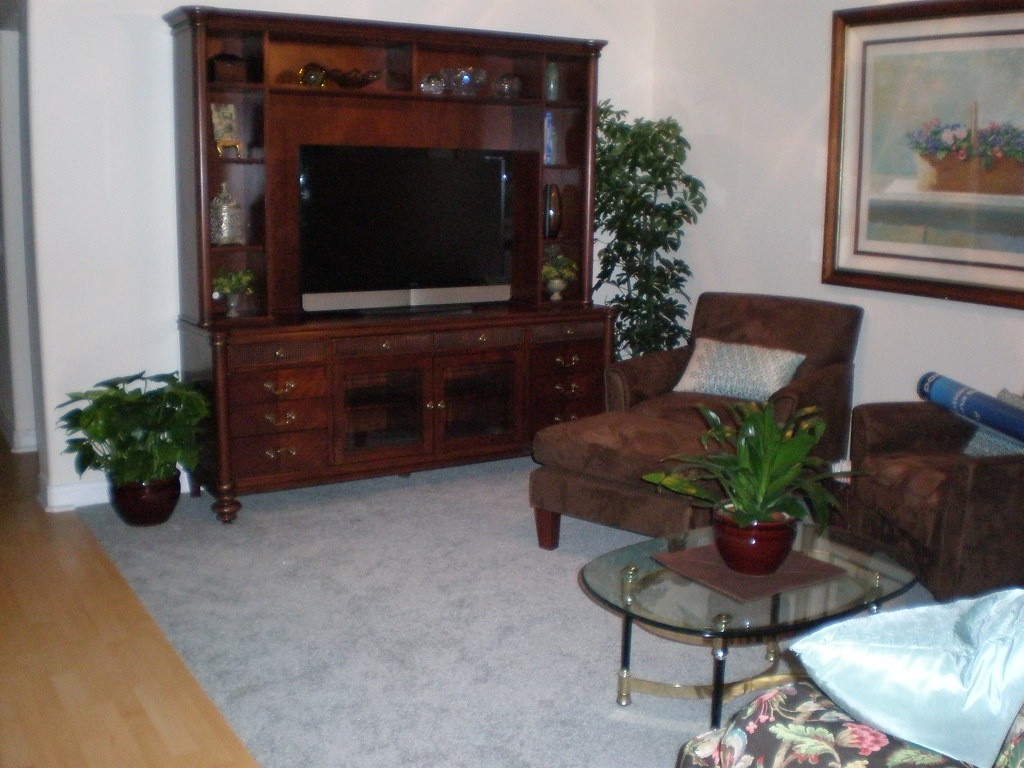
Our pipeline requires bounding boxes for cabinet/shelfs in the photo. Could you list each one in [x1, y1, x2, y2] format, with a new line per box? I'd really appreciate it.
[162, 4, 616, 499]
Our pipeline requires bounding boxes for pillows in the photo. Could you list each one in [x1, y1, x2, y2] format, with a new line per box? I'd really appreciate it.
[675, 334, 808, 405]
[963, 386, 1024, 456]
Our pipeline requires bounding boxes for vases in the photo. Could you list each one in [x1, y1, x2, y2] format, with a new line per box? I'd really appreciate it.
[224, 294, 241, 318]
[548, 278, 567, 301]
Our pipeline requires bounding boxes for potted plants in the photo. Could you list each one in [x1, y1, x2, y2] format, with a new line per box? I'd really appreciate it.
[59, 369, 210, 525]
[638, 399, 874, 577]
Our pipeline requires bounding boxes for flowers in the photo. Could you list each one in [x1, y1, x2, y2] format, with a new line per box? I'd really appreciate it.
[541, 252, 579, 283]
[209, 268, 256, 302]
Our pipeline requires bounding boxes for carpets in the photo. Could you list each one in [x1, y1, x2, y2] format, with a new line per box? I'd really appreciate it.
[75, 455, 939, 768]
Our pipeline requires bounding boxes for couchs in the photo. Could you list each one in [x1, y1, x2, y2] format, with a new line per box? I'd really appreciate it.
[530, 294, 866, 550]
[679, 681, 1024, 768]
[843, 398, 1024, 600]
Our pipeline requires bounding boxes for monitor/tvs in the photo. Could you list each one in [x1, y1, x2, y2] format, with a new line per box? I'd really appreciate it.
[300, 149, 512, 317]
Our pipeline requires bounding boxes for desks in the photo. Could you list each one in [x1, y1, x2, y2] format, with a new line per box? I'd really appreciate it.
[582, 524, 921, 729]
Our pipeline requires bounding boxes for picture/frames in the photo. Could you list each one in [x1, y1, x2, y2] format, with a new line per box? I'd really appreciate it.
[820, 0, 1024, 310]
